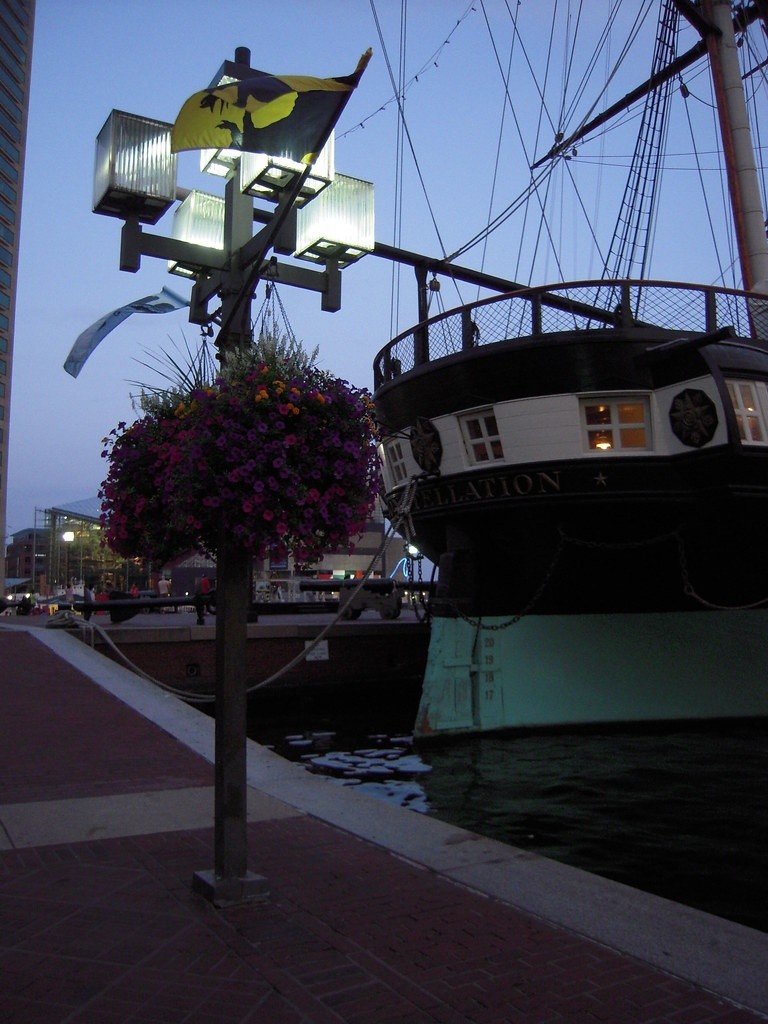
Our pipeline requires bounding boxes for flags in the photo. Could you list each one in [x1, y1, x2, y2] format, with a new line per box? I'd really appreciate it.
[171, 72, 360, 166]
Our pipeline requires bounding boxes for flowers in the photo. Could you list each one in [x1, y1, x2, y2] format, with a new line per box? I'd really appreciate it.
[93, 341, 393, 572]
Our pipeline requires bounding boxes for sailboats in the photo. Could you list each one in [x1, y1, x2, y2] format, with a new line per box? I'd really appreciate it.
[370, 0, 768, 743]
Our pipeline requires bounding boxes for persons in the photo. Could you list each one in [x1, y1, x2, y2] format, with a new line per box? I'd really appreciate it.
[344, 573, 350, 580]
[200, 574, 210, 615]
[30, 576, 171, 614]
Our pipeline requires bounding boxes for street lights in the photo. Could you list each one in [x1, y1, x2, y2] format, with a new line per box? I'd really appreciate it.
[93, 45, 376, 872]
